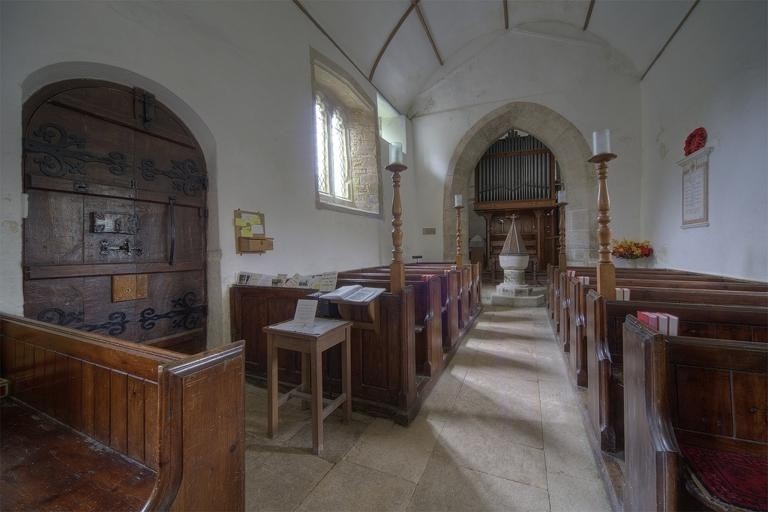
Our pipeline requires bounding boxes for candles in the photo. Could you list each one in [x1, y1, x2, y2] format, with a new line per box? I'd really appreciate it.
[455, 194, 464, 209]
[391, 146, 402, 163]
[591, 129, 610, 157]
[557, 190, 566, 204]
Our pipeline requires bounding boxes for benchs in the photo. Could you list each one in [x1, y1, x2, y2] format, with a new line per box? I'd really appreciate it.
[0, 305, 247, 512]
[230, 260, 482, 426]
[549, 266, 767, 510]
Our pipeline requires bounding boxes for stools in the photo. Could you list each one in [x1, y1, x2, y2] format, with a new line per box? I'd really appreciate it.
[260, 313, 355, 456]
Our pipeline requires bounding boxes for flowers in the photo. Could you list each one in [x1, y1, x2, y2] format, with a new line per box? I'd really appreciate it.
[608, 236, 655, 260]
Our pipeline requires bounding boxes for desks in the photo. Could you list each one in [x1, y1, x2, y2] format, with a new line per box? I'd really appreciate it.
[489, 253, 540, 286]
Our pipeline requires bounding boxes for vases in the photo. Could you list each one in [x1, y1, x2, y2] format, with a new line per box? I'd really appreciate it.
[621, 256, 649, 269]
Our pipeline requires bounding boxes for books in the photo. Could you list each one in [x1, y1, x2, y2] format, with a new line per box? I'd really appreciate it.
[637, 311, 678, 336]
[567, 270, 576, 277]
[319, 285, 386, 303]
[616, 288, 630, 301]
[577, 276, 589, 285]
